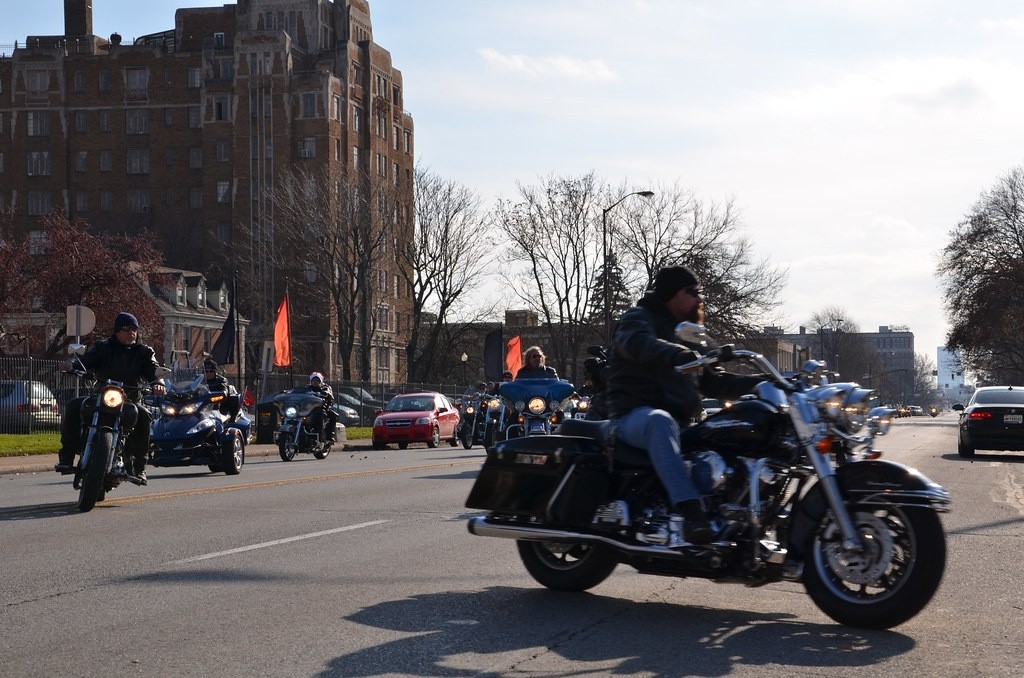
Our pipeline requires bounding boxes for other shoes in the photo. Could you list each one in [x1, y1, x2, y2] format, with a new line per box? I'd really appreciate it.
[56, 460, 73, 472]
[133, 459, 147, 483]
[683, 508, 713, 544]
[312, 441, 325, 452]
[326, 436, 336, 442]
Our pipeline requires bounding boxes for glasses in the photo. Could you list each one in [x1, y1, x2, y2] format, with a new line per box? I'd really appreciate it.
[532, 354, 542, 359]
[120, 324, 138, 332]
[205, 370, 213, 373]
[312, 378, 319, 382]
[683, 285, 702, 297]
[504, 375, 512, 379]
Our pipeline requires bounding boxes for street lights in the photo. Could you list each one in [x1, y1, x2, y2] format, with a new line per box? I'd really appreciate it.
[604, 191, 654, 350]
[820, 319, 844, 361]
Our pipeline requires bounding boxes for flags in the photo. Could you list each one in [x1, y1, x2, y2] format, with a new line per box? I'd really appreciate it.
[241, 387, 255, 406]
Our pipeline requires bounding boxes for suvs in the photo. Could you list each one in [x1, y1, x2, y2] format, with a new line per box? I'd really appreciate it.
[331, 387, 390, 410]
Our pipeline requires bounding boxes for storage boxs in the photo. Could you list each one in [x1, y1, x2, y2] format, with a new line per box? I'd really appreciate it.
[464, 434, 601, 520]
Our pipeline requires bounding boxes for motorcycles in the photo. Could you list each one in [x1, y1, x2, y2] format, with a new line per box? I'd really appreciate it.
[905, 407, 911, 417]
[569, 390, 594, 420]
[139, 349, 253, 475]
[930, 407, 937, 417]
[495, 378, 574, 442]
[59, 344, 172, 512]
[440, 322, 952, 628]
[895, 407, 907, 418]
[457, 388, 489, 450]
[272, 387, 332, 461]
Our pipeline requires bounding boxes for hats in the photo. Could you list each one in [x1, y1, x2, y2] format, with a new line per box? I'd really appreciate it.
[202, 360, 219, 373]
[113, 312, 139, 332]
[652, 266, 698, 304]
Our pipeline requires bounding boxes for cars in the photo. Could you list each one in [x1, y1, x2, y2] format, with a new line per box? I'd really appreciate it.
[908, 406, 922, 416]
[952, 386, 1024, 457]
[333, 392, 383, 425]
[0, 380, 62, 427]
[259, 391, 361, 428]
[732, 395, 757, 406]
[372, 392, 460, 449]
[701, 398, 722, 415]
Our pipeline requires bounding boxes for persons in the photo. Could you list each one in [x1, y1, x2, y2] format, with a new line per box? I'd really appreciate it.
[474, 346, 604, 445]
[605, 265, 783, 544]
[55, 311, 166, 486]
[194, 359, 229, 412]
[280, 372, 338, 447]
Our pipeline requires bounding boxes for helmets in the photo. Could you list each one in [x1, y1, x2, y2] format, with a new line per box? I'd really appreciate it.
[309, 372, 323, 386]
[503, 371, 513, 376]
